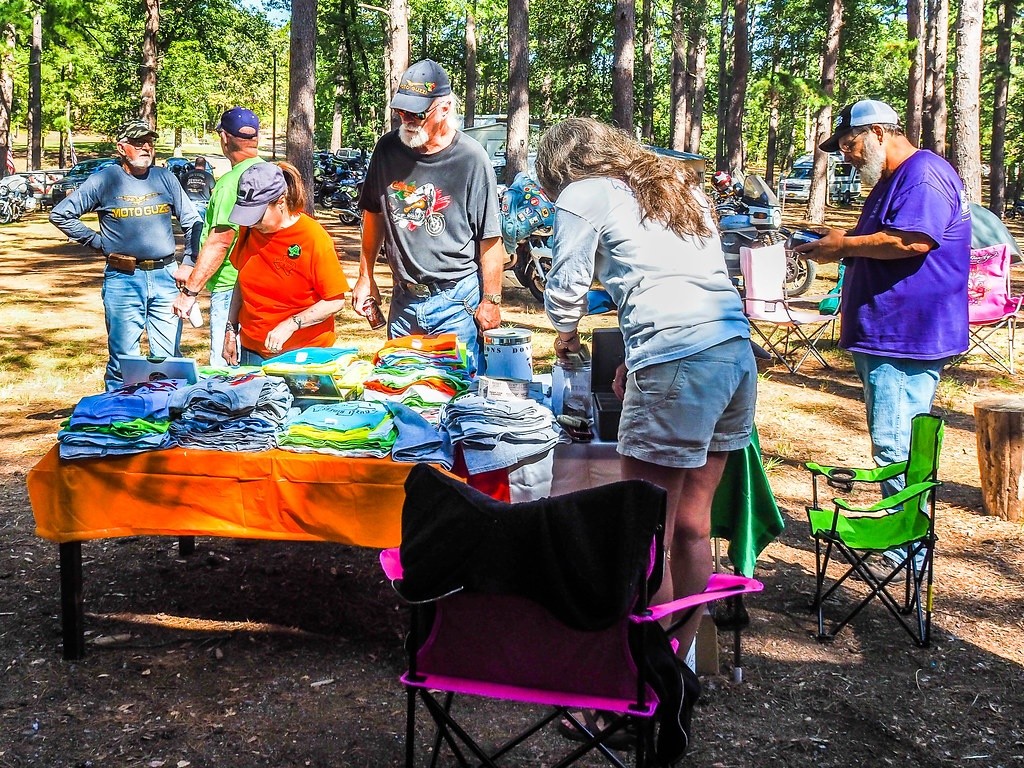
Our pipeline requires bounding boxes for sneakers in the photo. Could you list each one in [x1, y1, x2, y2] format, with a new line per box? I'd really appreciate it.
[846, 547, 926, 584]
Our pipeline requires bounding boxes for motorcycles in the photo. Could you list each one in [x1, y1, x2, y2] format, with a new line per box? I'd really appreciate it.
[706, 167, 817, 295]
[496, 168, 556, 302]
[0, 173, 35, 224]
[312, 148, 371, 226]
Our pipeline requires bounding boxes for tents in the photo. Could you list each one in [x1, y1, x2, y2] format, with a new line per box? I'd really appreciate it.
[967, 204, 1024, 265]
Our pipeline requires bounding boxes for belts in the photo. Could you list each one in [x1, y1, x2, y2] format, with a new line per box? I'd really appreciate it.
[392, 273, 465, 299]
[105, 253, 176, 271]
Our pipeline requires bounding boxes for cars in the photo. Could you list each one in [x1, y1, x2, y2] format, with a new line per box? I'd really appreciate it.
[51, 158, 117, 208]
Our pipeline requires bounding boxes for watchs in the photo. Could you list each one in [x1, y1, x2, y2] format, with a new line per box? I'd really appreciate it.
[182, 286, 198, 297]
[482, 293, 502, 305]
[292, 315, 301, 330]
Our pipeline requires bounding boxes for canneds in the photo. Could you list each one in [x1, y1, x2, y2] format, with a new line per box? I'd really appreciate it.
[362, 298, 387, 330]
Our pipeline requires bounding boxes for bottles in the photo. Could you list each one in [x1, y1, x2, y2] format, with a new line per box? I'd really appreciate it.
[483, 327, 533, 382]
[550, 359, 598, 442]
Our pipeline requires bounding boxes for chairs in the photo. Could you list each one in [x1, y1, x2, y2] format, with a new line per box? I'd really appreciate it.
[739, 240, 839, 375]
[947, 244, 1024, 374]
[379, 463, 763, 768]
[805, 413, 945, 648]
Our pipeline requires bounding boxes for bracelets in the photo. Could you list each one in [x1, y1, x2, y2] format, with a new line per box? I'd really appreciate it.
[226, 322, 238, 334]
[558, 329, 579, 345]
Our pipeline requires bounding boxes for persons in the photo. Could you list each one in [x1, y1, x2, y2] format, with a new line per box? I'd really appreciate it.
[221, 162, 350, 367]
[793, 100, 971, 586]
[544, 118, 756, 750]
[351, 59, 504, 377]
[49, 121, 204, 392]
[182, 157, 216, 220]
[172, 107, 267, 365]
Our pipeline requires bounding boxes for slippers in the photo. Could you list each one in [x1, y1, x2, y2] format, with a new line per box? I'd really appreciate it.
[557, 708, 637, 749]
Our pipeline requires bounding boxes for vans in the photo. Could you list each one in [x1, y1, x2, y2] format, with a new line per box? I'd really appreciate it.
[779, 154, 861, 206]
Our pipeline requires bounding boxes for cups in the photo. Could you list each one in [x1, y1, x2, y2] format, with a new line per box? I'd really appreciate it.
[188, 302, 204, 329]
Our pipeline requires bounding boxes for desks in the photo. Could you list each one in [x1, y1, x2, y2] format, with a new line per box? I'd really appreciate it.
[25, 423, 786, 685]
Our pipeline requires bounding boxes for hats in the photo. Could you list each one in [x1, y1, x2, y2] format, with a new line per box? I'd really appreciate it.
[215, 107, 259, 139]
[228, 162, 288, 226]
[818, 99, 898, 152]
[389, 58, 452, 113]
[115, 118, 159, 142]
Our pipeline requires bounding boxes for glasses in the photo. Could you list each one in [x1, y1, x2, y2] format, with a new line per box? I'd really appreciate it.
[393, 99, 445, 120]
[121, 137, 158, 149]
[840, 127, 870, 154]
